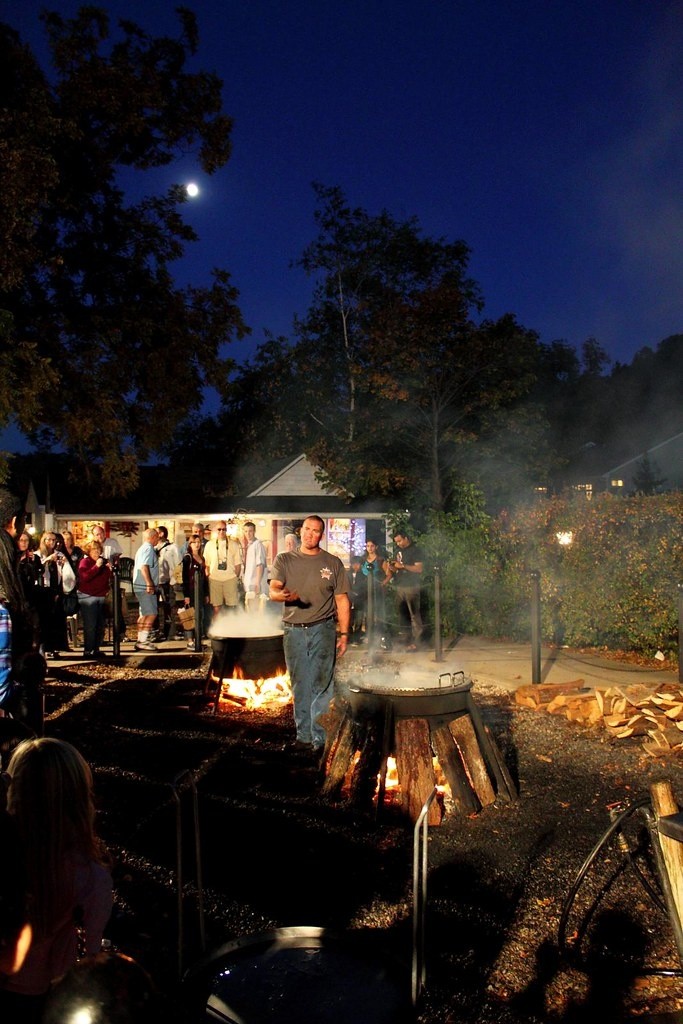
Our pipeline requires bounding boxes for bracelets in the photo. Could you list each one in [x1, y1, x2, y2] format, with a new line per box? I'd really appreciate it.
[340, 633, 350, 636]
[402, 564, 405, 568]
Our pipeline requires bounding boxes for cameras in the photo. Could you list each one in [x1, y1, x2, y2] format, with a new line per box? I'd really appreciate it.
[56, 556, 63, 560]
[389, 561, 395, 566]
[218, 562, 227, 570]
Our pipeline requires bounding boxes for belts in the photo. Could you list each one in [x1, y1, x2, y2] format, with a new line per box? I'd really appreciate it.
[286, 617, 331, 627]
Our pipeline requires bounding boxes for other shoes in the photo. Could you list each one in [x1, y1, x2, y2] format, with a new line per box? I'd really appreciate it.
[83, 652, 96, 659]
[56, 648, 73, 652]
[406, 642, 420, 652]
[135, 641, 158, 653]
[186, 642, 208, 651]
[282, 740, 312, 753]
[45, 652, 59, 660]
[93, 651, 105, 657]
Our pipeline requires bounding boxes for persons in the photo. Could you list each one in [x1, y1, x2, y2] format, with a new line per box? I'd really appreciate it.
[347, 531, 425, 652]
[267, 515, 351, 753]
[0, 491, 302, 656]
[0, 529, 119, 995]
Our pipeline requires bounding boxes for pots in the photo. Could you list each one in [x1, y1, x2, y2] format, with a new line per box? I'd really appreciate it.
[207, 630, 287, 680]
[346, 664, 474, 760]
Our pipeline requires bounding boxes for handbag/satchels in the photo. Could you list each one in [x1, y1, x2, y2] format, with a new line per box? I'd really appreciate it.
[177, 603, 196, 630]
